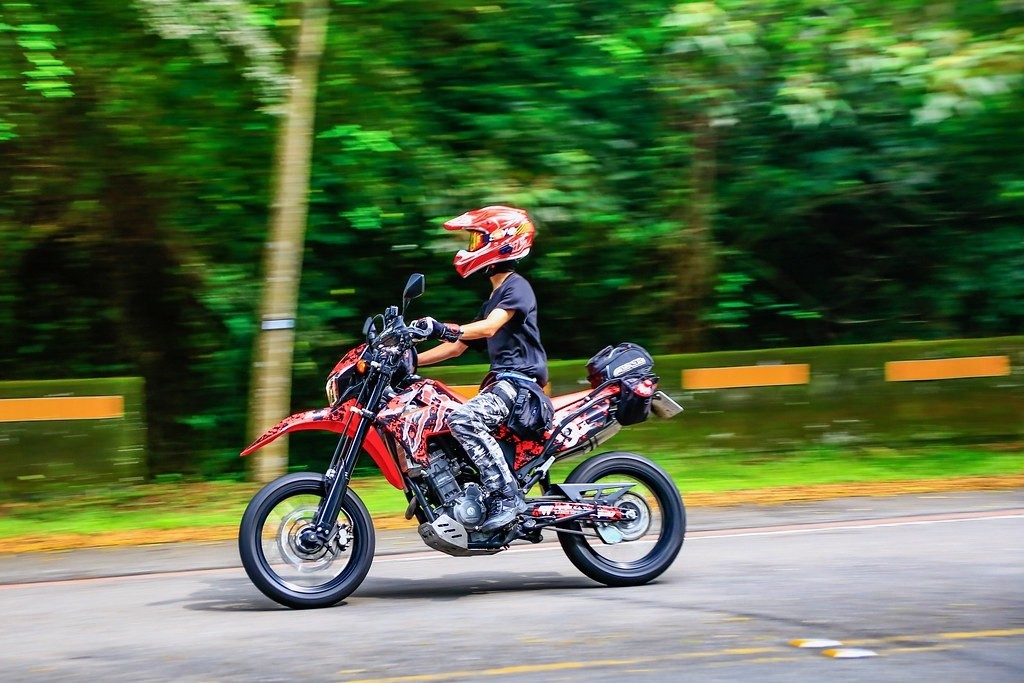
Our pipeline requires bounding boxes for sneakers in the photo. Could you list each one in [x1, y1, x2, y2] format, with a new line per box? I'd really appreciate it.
[481, 484, 528, 533]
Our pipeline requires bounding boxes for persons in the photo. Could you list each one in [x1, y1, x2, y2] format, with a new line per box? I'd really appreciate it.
[407, 205, 549, 532]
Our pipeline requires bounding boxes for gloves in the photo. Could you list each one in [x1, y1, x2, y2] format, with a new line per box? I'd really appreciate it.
[409, 316, 461, 344]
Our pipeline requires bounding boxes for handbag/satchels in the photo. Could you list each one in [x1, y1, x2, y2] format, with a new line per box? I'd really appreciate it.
[585, 343, 654, 388]
[506, 387, 555, 439]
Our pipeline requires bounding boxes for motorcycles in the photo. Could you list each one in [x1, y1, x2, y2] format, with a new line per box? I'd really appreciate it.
[238, 273, 687, 610]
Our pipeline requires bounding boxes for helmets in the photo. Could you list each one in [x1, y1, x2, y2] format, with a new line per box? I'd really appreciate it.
[443, 205, 535, 278]
